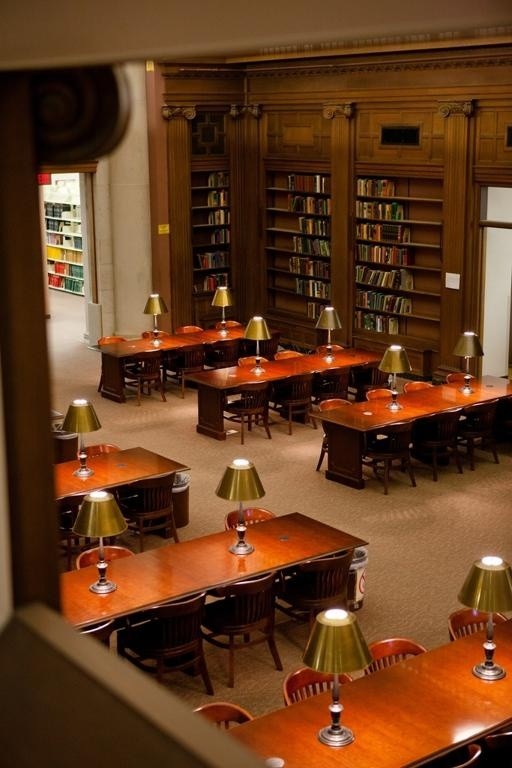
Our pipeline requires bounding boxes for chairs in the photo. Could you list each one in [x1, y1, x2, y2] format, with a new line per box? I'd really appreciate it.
[403, 381, 434, 393]
[283, 665, 354, 707]
[446, 373, 476, 385]
[448, 608, 508, 642]
[238, 356, 269, 367]
[60, 490, 116, 550]
[316, 345, 344, 354]
[78, 619, 115, 648]
[316, 399, 353, 473]
[76, 545, 135, 570]
[455, 398, 500, 470]
[255, 374, 317, 435]
[484, 732, 512, 752]
[222, 380, 272, 445]
[274, 351, 304, 361]
[363, 418, 417, 494]
[175, 326, 203, 335]
[142, 331, 169, 340]
[319, 365, 352, 399]
[364, 360, 384, 385]
[117, 591, 214, 696]
[414, 408, 463, 482]
[225, 508, 277, 532]
[274, 549, 354, 659]
[193, 702, 254, 732]
[124, 349, 167, 406]
[216, 321, 241, 329]
[364, 637, 429, 676]
[116, 472, 181, 552]
[201, 570, 283, 688]
[211, 338, 241, 369]
[454, 743, 482, 767]
[162, 343, 207, 399]
[365, 389, 397, 402]
[80, 444, 121, 459]
[97, 337, 127, 393]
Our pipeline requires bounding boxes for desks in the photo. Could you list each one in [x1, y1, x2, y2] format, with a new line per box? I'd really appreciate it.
[224, 617, 512, 768]
[56, 447, 192, 539]
[60, 512, 370, 643]
[308, 375, 512, 490]
[88, 325, 284, 404]
[183, 349, 384, 441]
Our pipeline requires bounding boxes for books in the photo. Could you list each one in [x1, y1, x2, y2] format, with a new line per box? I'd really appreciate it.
[210, 228, 231, 245]
[295, 277, 330, 301]
[304, 300, 330, 318]
[353, 309, 399, 336]
[291, 195, 331, 215]
[46, 245, 84, 266]
[208, 189, 229, 208]
[45, 218, 84, 237]
[355, 264, 414, 291]
[45, 232, 85, 251]
[354, 287, 413, 316]
[292, 235, 331, 257]
[202, 272, 230, 291]
[289, 255, 329, 280]
[47, 257, 84, 279]
[208, 209, 231, 225]
[356, 222, 411, 245]
[356, 179, 395, 198]
[287, 174, 331, 194]
[357, 243, 411, 266]
[298, 216, 331, 236]
[355, 200, 404, 221]
[47, 271, 85, 295]
[196, 250, 230, 270]
[208, 171, 230, 187]
[44, 201, 82, 221]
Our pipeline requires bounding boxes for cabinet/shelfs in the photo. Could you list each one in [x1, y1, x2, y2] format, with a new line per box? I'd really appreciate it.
[44, 200, 86, 296]
[262, 167, 333, 348]
[190, 167, 232, 328]
[352, 173, 443, 380]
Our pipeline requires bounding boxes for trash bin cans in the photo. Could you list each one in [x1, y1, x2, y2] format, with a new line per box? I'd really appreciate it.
[350, 547, 369, 612]
[172, 472, 191, 528]
[53, 422, 78, 463]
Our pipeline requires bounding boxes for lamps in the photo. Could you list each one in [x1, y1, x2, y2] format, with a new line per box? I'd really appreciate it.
[453, 331, 484, 394]
[62, 399, 102, 478]
[143, 294, 168, 346]
[244, 316, 270, 374]
[458, 556, 512, 681]
[212, 287, 237, 334]
[378, 344, 412, 411]
[72, 491, 128, 594]
[302, 608, 374, 747]
[315, 307, 342, 362]
[215, 459, 266, 556]
